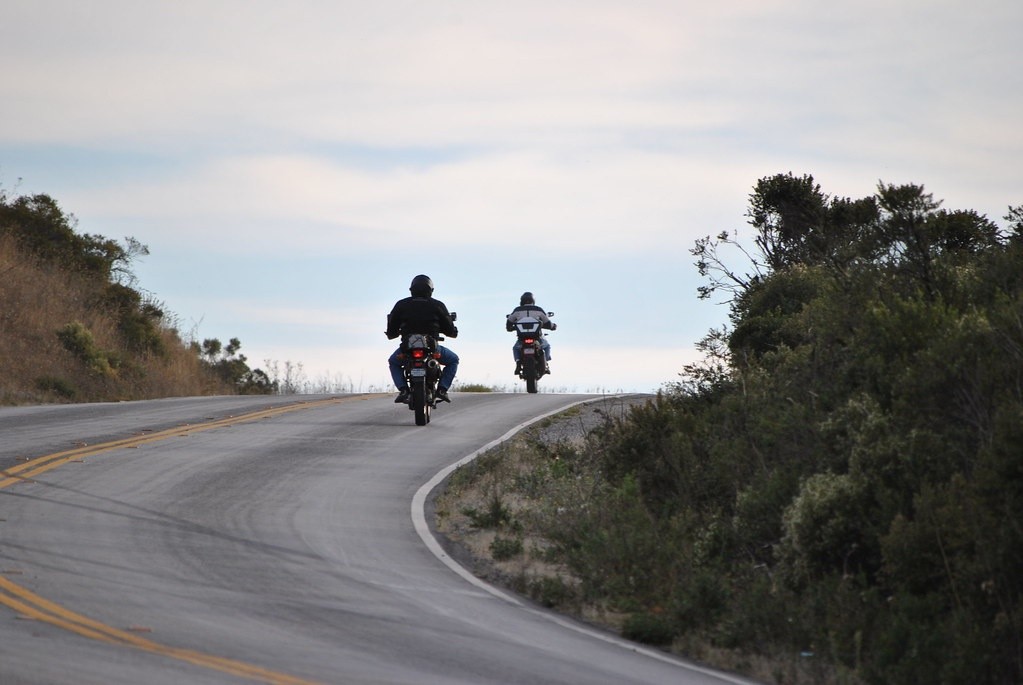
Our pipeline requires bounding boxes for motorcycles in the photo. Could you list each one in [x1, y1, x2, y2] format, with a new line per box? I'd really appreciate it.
[506, 311, 556, 392]
[384, 313, 457, 427]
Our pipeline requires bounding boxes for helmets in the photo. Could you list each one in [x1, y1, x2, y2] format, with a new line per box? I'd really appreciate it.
[520, 292, 535, 305]
[410, 275, 433, 297]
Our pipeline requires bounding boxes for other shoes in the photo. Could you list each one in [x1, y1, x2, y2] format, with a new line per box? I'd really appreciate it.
[546, 363, 550, 374]
[514, 362, 522, 375]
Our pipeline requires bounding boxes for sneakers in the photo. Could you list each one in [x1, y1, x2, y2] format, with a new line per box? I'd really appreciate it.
[436, 388, 451, 403]
[395, 387, 410, 403]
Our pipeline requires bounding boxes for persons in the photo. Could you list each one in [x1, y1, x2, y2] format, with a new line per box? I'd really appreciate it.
[387, 274, 460, 403]
[506, 292, 556, 374]
